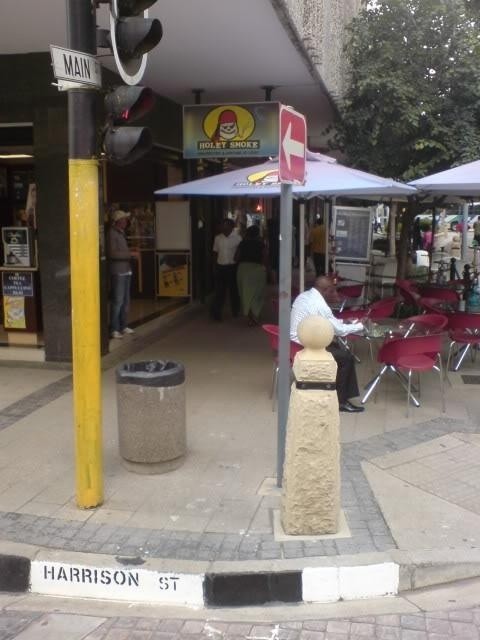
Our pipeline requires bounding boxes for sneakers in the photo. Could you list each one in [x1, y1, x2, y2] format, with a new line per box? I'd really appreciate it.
[112, 327, 135, 338]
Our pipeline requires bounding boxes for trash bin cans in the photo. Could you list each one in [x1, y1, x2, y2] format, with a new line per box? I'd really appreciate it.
[113, 360, 189, 471]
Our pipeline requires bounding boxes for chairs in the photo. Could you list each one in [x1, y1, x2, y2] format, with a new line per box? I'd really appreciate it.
[261, 269, 478, 418]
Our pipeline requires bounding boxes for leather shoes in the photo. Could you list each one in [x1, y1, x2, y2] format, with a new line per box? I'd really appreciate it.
[340, 401, 364, 412]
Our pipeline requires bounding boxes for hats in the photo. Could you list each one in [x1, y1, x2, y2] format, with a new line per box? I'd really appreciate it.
[113, 210, 132, 222]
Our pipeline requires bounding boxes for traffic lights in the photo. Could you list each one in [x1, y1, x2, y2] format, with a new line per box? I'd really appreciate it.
[115, 0, 163, 59]
[101, 84, 155, 170]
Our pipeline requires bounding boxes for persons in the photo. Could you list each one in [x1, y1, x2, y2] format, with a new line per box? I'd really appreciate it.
[107, 210, 135, 339]
[212, 219, 243, 320]
[289, 276, 364, 412]
[234, 225, 267, 327]
